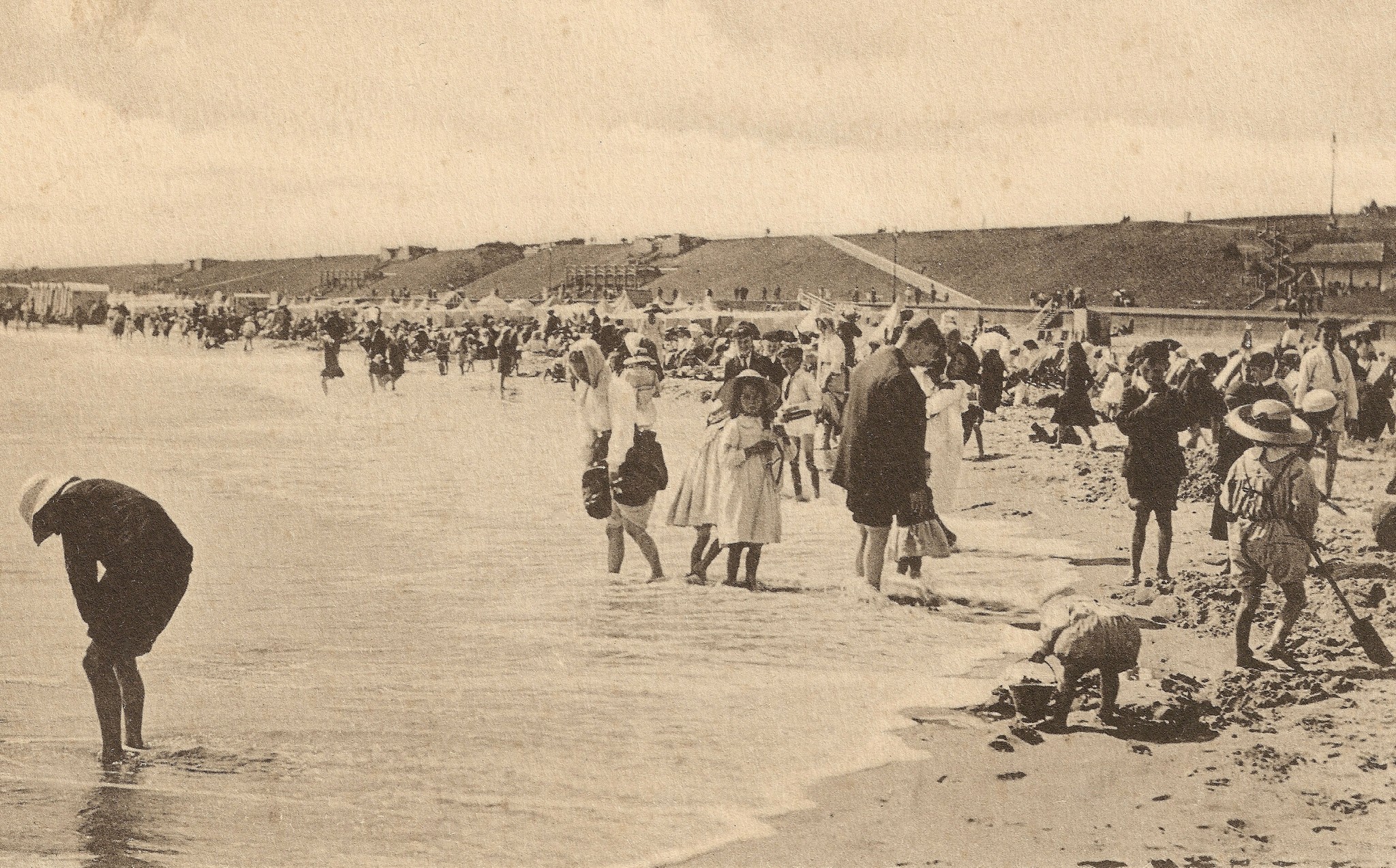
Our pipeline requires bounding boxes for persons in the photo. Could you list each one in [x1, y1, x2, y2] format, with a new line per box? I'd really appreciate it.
[15, 476, 193, 756]
[0, 286, 1396, 735]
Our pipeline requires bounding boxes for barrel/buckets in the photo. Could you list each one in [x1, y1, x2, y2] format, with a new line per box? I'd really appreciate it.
[1006, 656, 1057, 722]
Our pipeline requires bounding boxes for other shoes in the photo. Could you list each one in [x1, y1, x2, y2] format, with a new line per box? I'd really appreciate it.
[795, 495, 809, 502]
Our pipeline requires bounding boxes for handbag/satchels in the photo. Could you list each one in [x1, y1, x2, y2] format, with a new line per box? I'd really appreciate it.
[582, 467, 611, 519]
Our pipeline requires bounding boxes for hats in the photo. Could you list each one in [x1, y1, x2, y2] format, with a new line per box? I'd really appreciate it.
[718, 370, 780, 411]
[18, 475, 83, 546]
[816, 316, 835, 333]
[1226, 400, 1312, 443]
[1039, 587, 1076, 605]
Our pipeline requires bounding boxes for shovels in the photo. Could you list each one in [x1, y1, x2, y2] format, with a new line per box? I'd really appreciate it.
[1310, 542, 1396, 665]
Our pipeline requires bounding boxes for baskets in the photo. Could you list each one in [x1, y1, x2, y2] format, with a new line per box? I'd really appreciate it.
[1006, 657, 1058, 722]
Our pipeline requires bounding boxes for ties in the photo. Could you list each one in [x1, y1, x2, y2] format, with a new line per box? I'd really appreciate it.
[1322, 344, 1340, 383]
[740, 354, 749, 369]
[784, 372, 796, 399]
